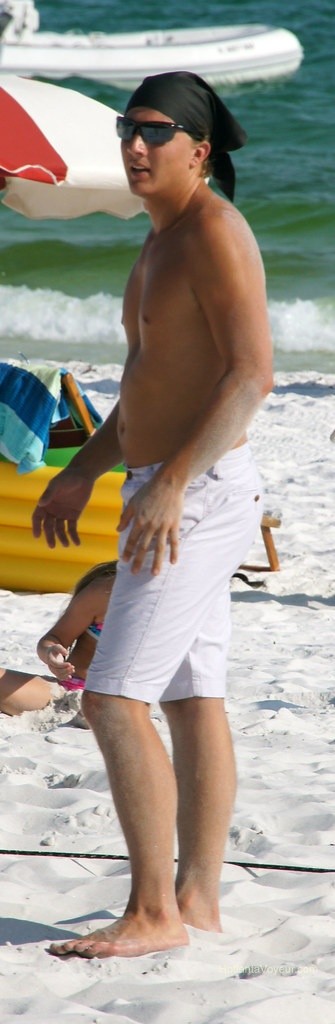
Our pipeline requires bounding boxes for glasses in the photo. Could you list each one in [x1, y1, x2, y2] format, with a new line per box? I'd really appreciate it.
[116, 116, 205, 146]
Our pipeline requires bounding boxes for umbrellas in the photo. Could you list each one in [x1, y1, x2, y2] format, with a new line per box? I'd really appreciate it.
[1, 71, 214, 224]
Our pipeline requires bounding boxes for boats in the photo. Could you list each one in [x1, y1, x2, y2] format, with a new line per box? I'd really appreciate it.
[0, 0, 304, 94]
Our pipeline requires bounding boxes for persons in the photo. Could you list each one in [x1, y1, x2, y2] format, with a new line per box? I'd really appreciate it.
[0, 558, 120, 731]
[32, 71, 273, 958]
